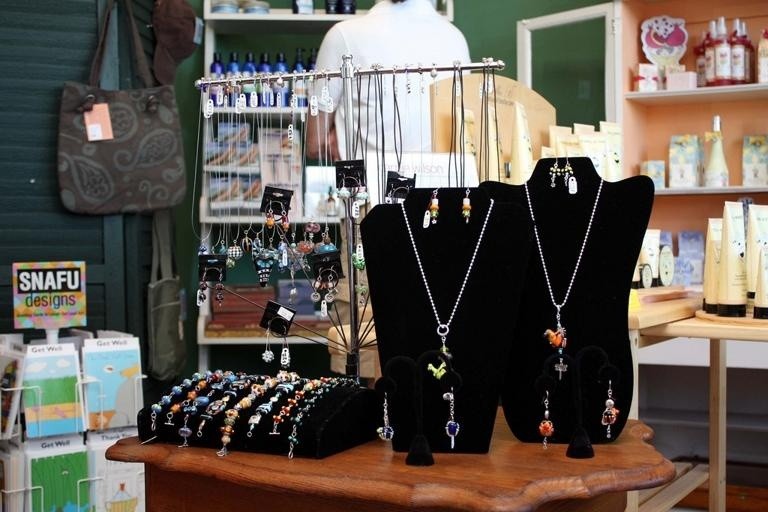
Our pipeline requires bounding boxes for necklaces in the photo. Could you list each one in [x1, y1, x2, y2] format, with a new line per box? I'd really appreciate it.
[188, 55, 505, 305]
[399, 199, 495, 380]
[524, 177, 606, 385]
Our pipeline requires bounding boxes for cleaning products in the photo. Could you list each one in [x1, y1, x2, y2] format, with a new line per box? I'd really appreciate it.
[210, 48, 316, 107]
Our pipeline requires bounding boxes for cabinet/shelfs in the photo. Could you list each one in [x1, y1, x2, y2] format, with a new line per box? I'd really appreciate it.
[201, 7, 455, 341]
[617, 0, 768, 294]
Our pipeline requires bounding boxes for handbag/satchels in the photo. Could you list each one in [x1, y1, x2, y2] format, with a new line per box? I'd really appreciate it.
[146, 211, 190, 382]
[56, 0, 187, 216]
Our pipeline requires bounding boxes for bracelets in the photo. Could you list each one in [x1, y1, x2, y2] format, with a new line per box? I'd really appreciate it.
[148, 365, 361, 462]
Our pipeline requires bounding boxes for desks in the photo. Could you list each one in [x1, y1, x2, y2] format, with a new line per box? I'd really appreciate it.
[104, 405, 673, 512]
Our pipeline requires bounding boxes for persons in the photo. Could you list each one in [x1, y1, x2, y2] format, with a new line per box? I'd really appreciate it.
[304, 0, 472, 290]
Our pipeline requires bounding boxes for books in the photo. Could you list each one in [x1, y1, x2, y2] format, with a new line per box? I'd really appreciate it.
[0, 328, 146, 512]
[205, 280, 335, 341]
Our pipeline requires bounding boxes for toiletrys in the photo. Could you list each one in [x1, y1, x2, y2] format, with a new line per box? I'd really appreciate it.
[631, 224, 677, 289]
[702, 199, 767, 321]
[454, 99, 624, 192]
[701, 200, 767, 318]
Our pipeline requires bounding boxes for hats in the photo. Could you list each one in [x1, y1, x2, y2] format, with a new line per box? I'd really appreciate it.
[151, 1, 204, 85]
